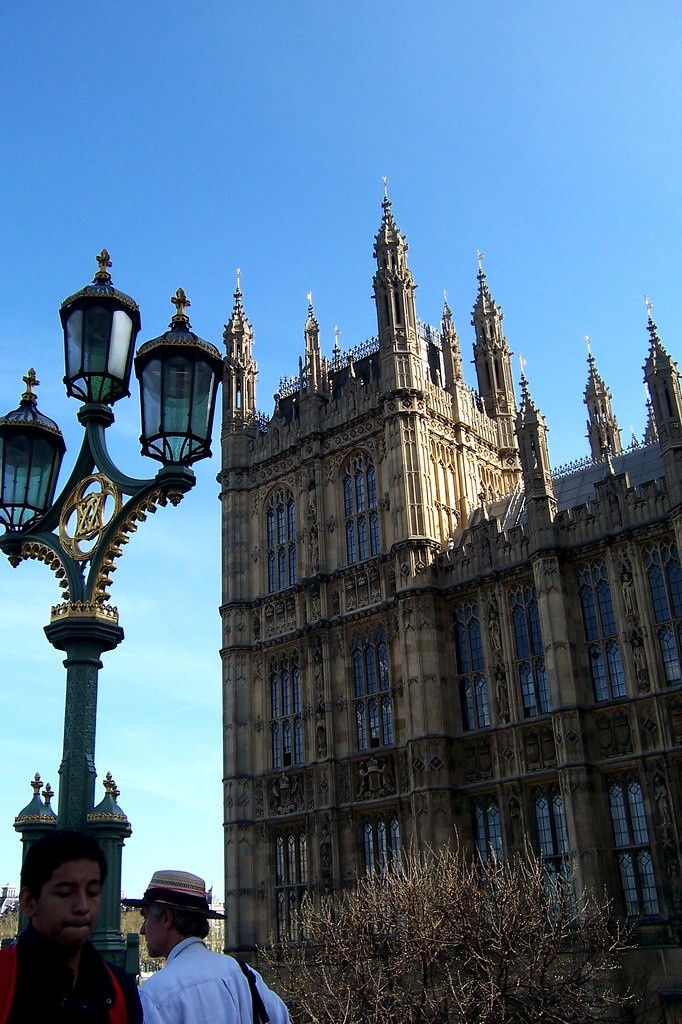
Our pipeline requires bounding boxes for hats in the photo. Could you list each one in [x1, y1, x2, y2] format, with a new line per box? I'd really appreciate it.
[122, 870, 227, 919]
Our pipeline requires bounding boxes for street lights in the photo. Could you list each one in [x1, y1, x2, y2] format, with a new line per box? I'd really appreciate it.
[0, 250, 222, 981]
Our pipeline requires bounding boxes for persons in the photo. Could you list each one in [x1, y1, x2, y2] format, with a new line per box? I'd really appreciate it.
[0, 830, 143, 1024]
[120, 870, 292, 1024]
